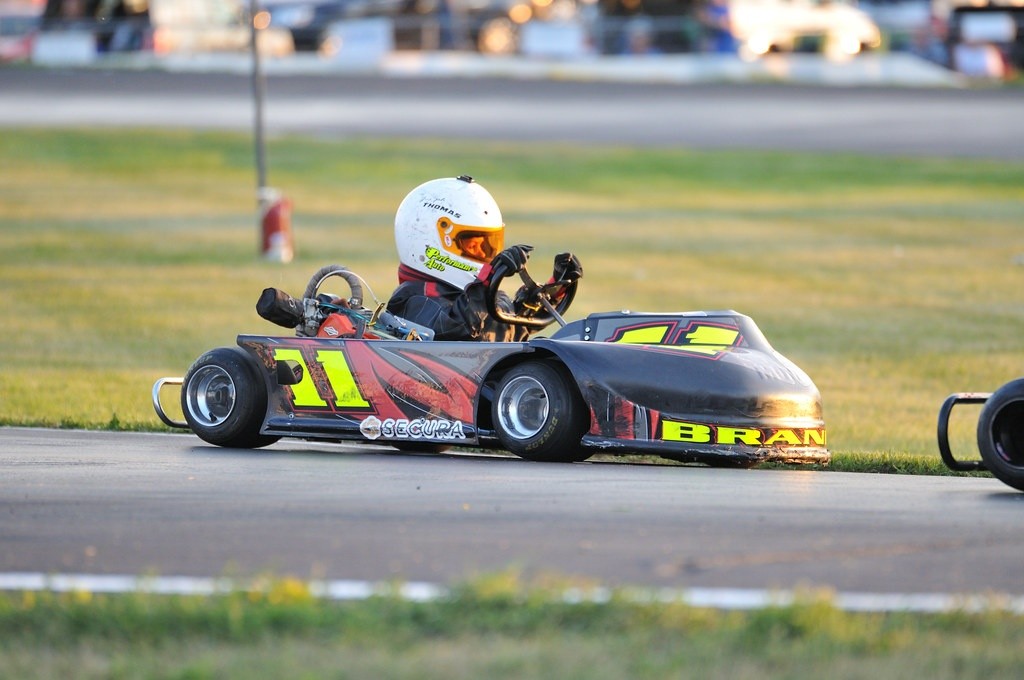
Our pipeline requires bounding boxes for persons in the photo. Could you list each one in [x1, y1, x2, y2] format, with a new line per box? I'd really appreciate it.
[375, 172, 583, 345]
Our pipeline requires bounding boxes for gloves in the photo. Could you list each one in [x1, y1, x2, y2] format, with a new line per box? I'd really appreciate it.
[553, 252, 583, 288]
[491, 244, 534, 276]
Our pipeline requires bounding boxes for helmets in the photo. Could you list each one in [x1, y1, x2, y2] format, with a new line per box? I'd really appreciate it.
[394, 174, 505, 292]
